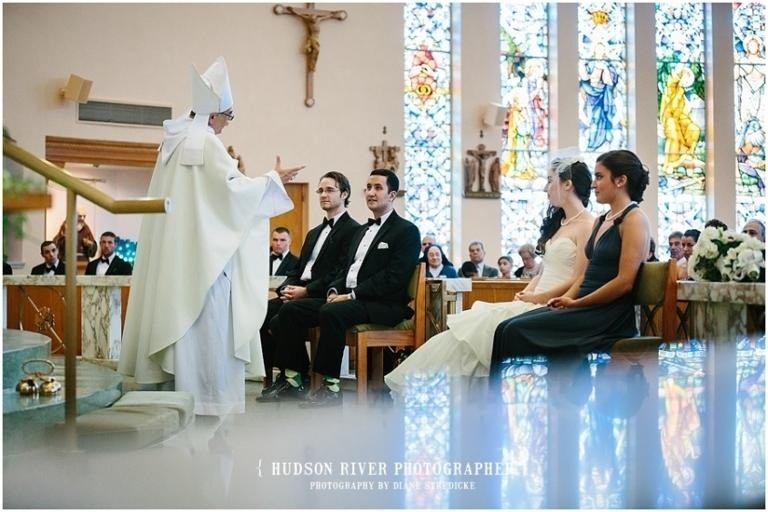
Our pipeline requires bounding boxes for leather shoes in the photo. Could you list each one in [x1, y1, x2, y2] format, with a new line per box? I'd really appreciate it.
[261, 374, 307, 400]
[298, 385, 343, 409]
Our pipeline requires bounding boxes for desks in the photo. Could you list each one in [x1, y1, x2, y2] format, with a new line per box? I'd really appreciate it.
[3, 273, 134, 360]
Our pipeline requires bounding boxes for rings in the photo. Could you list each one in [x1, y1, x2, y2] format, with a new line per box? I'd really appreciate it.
[291, 176, 294, 180]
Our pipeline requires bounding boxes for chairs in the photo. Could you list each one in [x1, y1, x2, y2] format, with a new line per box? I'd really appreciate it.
[297, 259, 426, 414]
[609, 257, 679, 353]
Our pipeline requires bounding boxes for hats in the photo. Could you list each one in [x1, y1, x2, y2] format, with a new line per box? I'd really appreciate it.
[188, 55, 233, 115]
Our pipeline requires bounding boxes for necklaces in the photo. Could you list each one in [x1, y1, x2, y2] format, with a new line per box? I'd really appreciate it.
[559, 209, 585, 226]
[602, 198, 638, 222]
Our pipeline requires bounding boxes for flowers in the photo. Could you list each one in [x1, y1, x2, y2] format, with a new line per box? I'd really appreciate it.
[686, 222, 768, 286]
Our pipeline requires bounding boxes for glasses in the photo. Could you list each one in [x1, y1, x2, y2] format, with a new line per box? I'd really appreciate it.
[317, 188, 339, 193]
[222, 111, 236, 121]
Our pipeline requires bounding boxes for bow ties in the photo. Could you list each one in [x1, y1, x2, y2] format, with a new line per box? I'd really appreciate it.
[272, 254, 282, 260]
[46, 265, 56, 272]
[323, 217, 334, 228]
[101, 258, 109, 265]
[368, 218, 381, 226]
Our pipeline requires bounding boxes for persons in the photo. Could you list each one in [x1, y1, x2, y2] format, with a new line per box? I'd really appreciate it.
[84, 230, 134, 277]
[542, 353, 650, 421]
[52, 206, 98, 264]
[378, 145, 597, 408]
[293, 169, 426, 411]
[28, 240, 79, 276]
[639, 213, 765, 337]
[732, 37, 765, 132]
[286, 6, 336, 75]
[253, 170, 363, 403]
[418, 234, 542, 285]
[463, 151, 501, 193]
[578, 41, 623, 153]
[268, 226, 300, 281]
[114, 104, 309, 425]
[485, 149, 662, 401]
[659, 50, 705, 180]
